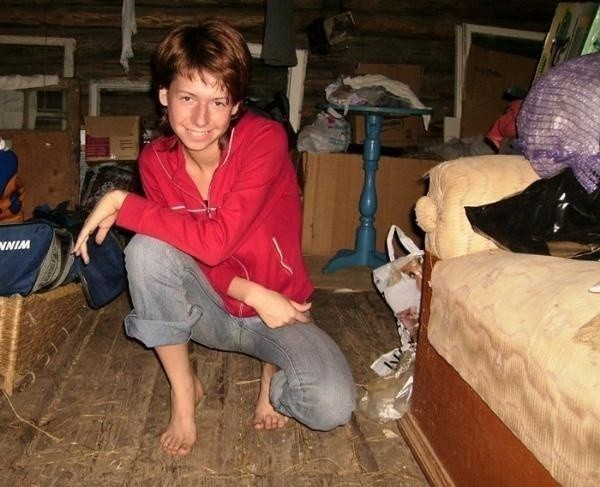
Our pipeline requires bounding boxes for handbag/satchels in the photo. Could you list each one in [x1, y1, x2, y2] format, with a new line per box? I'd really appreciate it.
[370, 225, 433, 381]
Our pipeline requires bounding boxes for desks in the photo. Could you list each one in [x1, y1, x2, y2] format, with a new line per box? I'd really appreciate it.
[314, 95, 433, 277]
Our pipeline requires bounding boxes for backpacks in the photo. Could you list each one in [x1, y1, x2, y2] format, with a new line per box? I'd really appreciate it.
[0, 216, 79, 294]
[77, 159, 142, 214]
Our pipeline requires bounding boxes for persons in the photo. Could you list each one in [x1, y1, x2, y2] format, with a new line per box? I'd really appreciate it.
[67, 17, 358, 457]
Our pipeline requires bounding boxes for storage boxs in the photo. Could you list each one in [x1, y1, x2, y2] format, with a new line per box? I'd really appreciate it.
[84, 115, 140, 161]
[300, 61, 447, 258]
